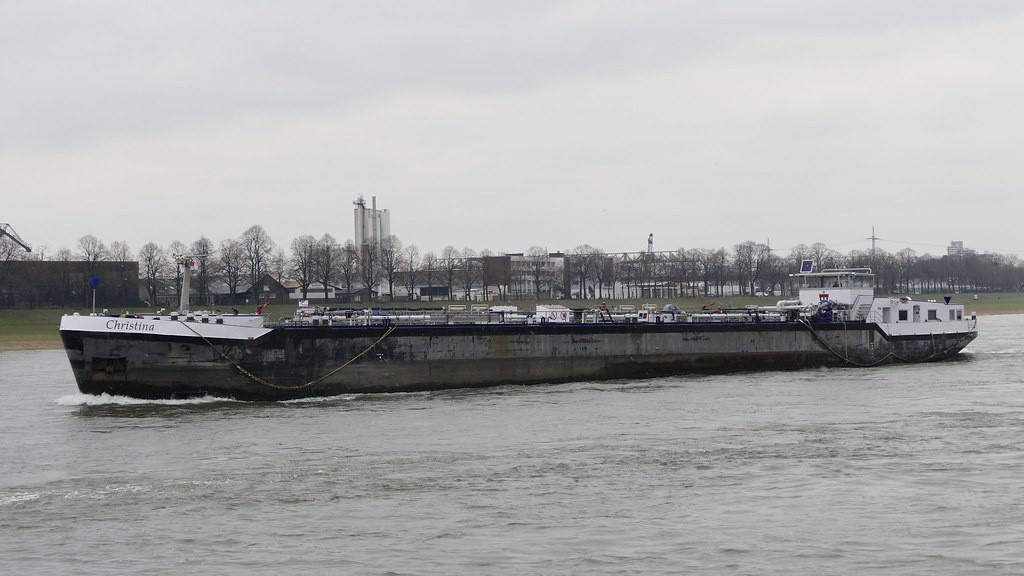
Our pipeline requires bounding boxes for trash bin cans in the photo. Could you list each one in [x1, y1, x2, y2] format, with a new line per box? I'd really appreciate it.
[632, 314, 639, 325]
[624, 314, 631, 324]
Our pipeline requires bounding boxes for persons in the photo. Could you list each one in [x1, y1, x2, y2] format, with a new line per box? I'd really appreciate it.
[231, 307, 239, 316]
[601, 302, 608, 310]
[833, 281, 839, 287]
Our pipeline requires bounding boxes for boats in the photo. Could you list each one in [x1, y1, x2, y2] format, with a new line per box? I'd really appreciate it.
[60, 260, 979, 400]
